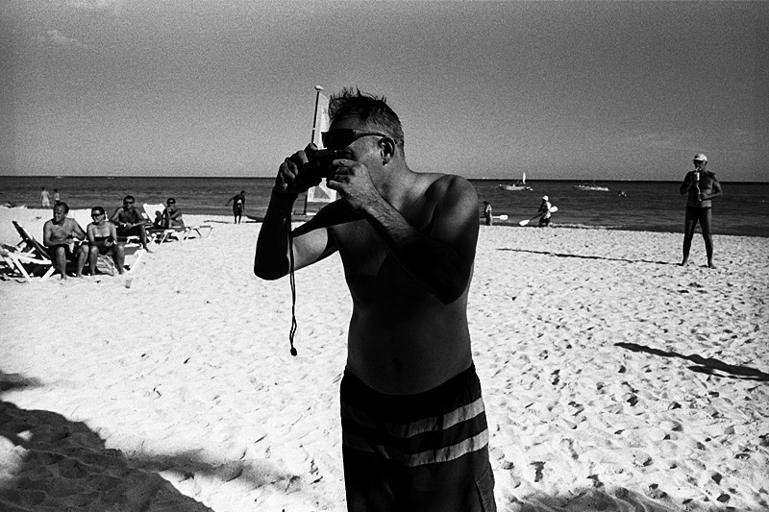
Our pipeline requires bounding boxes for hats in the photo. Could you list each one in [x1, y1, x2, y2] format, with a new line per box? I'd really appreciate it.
[542, 195, 548, 201]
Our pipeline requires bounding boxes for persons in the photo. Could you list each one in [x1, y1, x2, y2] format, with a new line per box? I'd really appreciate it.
[253, 82, 499, 511]
[43, 201, 91, 279]
[482, 199, 494, 227]
[39, 186, 53, 209]
[51, 187, 60, 207]
[224, 189, 247, 225]
[87, 205, 125, 276]
[677, 150, 725, 272]
[538, 193, 552, 228]
[154, 196, 183, 243]
[109, 194, 153, 254]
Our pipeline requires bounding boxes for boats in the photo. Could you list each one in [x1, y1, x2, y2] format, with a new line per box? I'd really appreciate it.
[573, 183, 608, 191]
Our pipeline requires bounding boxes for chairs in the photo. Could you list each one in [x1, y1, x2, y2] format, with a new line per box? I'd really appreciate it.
[0, 203, 214, 281]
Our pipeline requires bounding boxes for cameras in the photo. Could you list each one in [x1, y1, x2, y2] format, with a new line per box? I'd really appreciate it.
[307, 148, 355, 179]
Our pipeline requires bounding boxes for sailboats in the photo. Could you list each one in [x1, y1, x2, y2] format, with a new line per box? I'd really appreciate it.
[499, 171, 530, 191]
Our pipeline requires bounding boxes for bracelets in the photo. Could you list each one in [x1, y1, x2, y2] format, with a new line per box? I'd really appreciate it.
[133, 223, 136, 227]
[112, 239, 117, 246]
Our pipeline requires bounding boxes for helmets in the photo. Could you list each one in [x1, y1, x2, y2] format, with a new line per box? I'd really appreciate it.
[694, 153, 707, 161]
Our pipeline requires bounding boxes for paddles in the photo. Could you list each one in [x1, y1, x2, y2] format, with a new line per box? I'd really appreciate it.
[519, 206, 558, 227]
[491, 214, 508, 221]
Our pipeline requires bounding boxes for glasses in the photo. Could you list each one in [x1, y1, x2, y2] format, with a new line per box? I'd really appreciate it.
[318, 126, 395, 147]
[91, 214, 99, 216]
[125, 201, 133, 204]
[169, 202, 174, 205]
